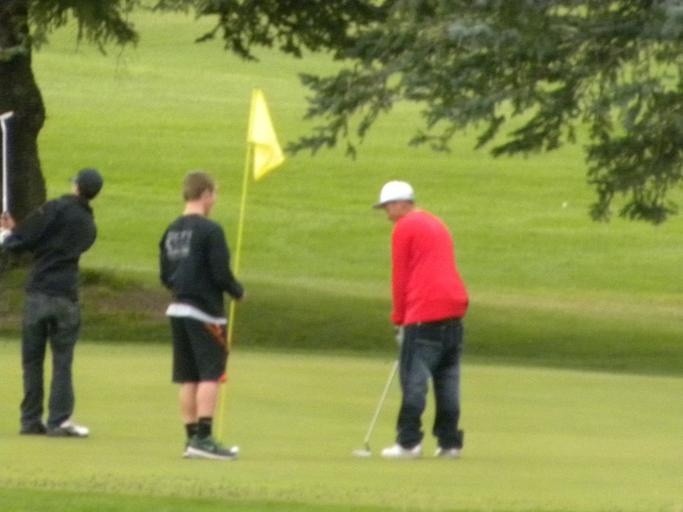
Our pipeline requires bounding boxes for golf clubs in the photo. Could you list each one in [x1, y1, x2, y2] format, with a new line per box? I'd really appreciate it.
[1, 111, 14, 214]
[362, 358, 398, 454]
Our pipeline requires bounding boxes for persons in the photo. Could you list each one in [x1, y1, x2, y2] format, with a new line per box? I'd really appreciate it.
[0, 168, 103, 435]
[373, 181, 469, 459]
[161, 172, 244, 461]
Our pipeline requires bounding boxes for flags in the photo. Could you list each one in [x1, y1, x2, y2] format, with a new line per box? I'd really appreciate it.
[248, 86, 286, 182]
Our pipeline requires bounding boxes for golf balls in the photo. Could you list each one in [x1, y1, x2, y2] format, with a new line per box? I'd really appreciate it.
[561, 202, 567, 207]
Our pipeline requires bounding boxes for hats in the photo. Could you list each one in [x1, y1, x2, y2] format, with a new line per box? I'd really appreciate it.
[372, 180, 414, 208]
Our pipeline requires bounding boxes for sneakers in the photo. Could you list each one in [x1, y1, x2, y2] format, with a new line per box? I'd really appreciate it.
[181, 435, 240, 461]
[380, 442, 422, 458]
[430, 446, 460, 459]
[19, 422, 89, 438]
